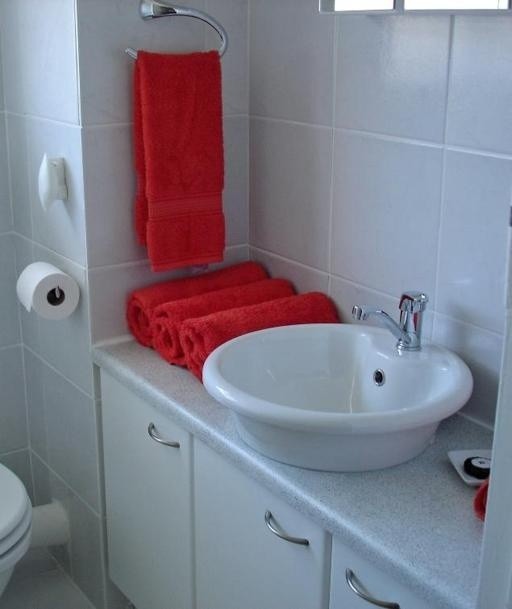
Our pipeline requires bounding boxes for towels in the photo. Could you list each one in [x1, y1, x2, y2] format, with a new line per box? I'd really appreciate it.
[125, 259, 268, 346]
[180, 291, 339, 384]
[133, 49, 226, 270]
[151, 277, 296, 373]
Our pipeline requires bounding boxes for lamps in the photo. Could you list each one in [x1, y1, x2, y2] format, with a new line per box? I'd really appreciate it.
[98, 367, 193, 609]
[193, 434, 330, 609]
[330, 530, 429, 609]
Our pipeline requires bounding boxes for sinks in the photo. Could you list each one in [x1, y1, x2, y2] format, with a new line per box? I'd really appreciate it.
[201, 322, 474, 473]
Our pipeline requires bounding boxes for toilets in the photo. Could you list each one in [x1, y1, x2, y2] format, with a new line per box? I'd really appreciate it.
[0, 461, 35, 601]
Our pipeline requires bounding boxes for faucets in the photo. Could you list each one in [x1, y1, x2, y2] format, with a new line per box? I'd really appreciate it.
[352, 290, 429, 352]
